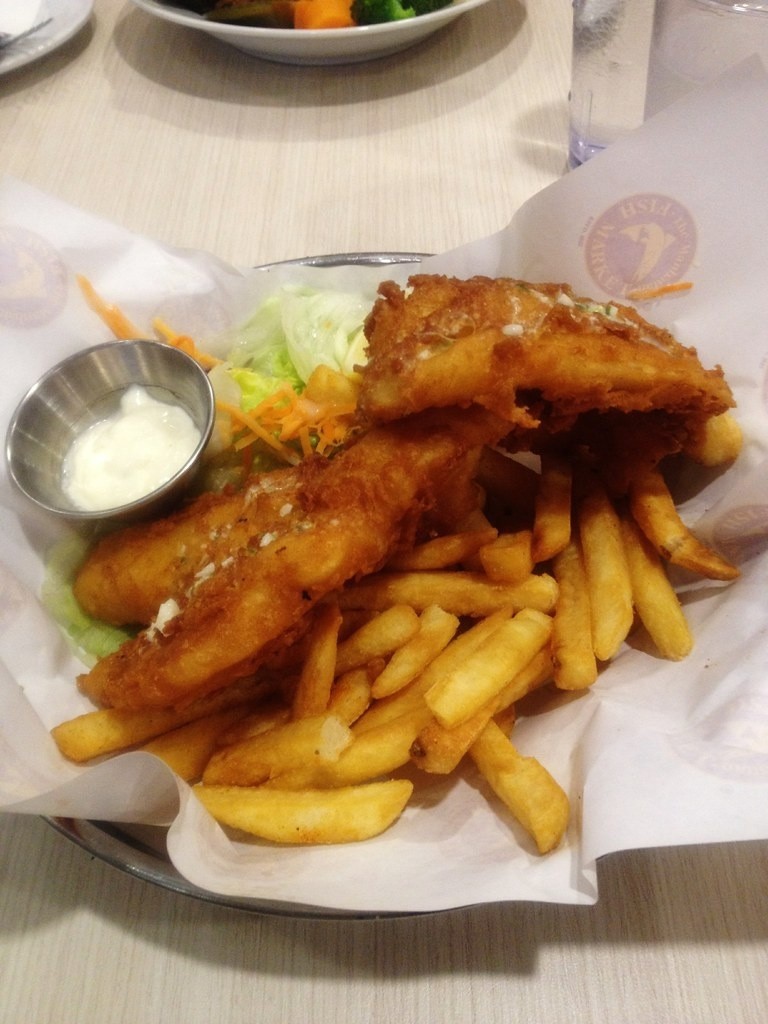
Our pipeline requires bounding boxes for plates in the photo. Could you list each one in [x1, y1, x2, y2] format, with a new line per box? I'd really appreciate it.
[0, 0, 94, 76]
[35, 249, 476, 923]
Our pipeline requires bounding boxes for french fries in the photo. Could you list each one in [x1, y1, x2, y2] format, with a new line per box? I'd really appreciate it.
[49, 407, 744, 858]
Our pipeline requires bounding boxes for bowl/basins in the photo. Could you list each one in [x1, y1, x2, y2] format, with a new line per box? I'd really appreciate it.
[129, 0, 490, 67]
[4, 338, 217, 526]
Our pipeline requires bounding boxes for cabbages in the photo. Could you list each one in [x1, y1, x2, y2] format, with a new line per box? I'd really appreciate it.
[197, 280, 376, 463]
[46, 519, 142, 662]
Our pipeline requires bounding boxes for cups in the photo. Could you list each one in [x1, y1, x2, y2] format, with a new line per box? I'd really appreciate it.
[565, 0, 768, 184]
